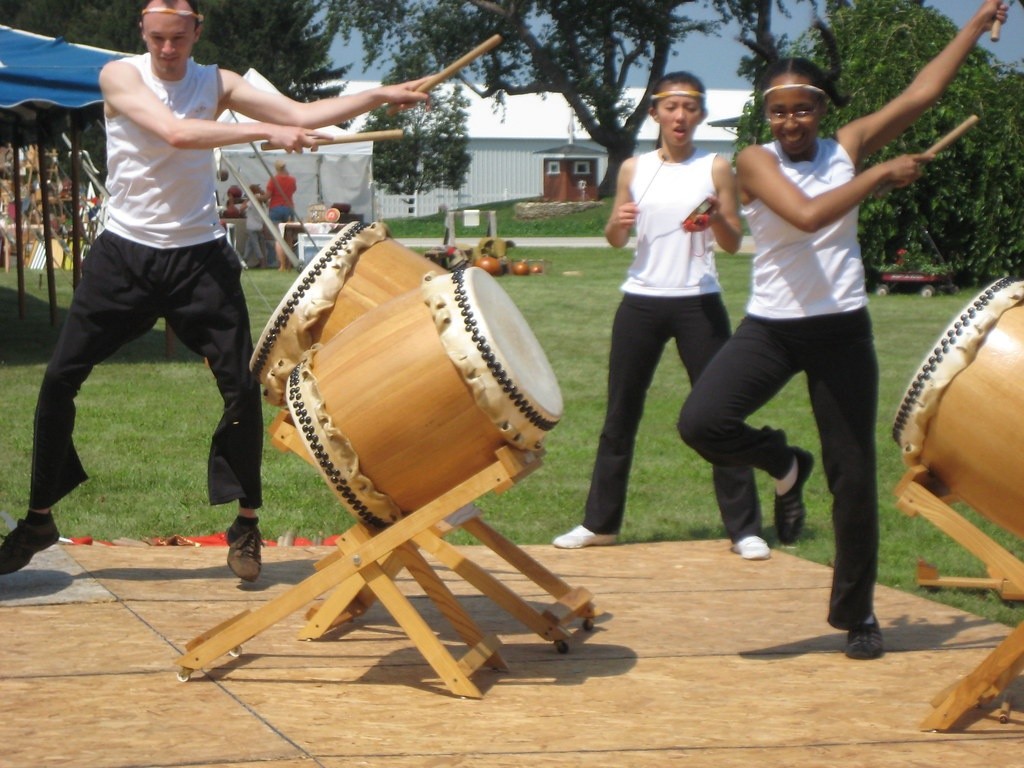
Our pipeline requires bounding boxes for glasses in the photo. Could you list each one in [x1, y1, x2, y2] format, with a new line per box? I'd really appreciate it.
[765, 100, 818, 124]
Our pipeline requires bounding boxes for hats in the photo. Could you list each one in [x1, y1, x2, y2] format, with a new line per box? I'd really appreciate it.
[274, 159, 285, 172]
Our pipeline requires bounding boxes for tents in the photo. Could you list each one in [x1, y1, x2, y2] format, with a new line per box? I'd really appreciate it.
[0, 23, 142, 327]
[212, 67, 375, 224]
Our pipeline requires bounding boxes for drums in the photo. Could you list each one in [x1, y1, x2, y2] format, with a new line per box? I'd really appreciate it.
[285, 267, 566, 535]
[249, 221, 455, 407]
[890, 275, 1024, 540]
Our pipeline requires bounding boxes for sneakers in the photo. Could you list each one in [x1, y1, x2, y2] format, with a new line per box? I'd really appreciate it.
[553, 526, 618, 548]
[774, 447, 814, 544]
[846, 613, 882, 659]
[226, 522, 264, 580]
[0, 515, 59, 574]
[733, 536, 770, 560]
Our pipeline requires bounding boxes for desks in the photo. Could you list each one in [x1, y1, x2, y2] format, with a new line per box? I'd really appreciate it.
[280, 222, 347, 272]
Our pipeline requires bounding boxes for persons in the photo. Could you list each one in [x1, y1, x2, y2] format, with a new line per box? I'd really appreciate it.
[0, 0, 437, 581]
[678, 0, 1008, 660]
[552, 71, 770, 559]
